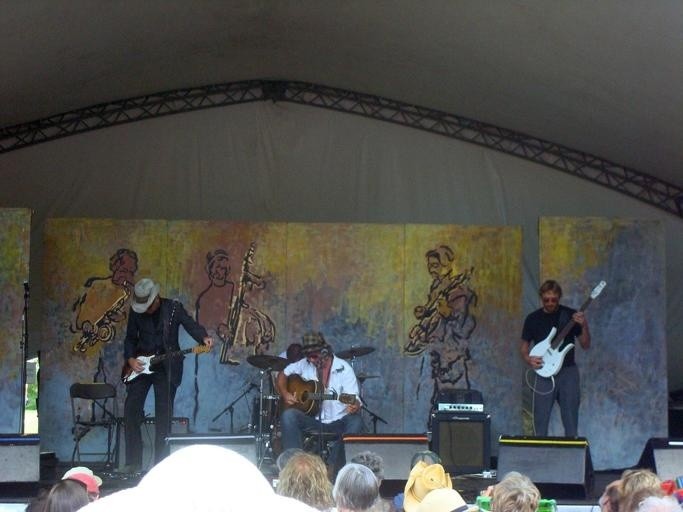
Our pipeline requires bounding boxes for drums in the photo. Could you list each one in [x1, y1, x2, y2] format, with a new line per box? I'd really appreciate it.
[252, 395, 281, 433]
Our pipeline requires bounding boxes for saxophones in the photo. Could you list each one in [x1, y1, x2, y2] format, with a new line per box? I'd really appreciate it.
[74, 280, 131, 353]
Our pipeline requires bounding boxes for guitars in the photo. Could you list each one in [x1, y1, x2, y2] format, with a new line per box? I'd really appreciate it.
[279, 373, 356, 415]
[121, 345, 209, 383]
[404, 268, 477, 356]
[529, 280, 607, 379]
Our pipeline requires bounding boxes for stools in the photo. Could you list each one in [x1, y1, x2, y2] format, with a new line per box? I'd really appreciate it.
[304, 430, 336, 466]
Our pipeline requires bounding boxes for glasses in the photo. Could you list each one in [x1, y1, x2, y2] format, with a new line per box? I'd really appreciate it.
[543, 297, 558, 303]
[305, 353, 320, 361]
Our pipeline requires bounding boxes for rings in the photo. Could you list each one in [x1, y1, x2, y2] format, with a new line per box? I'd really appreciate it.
[136, 359, 140, 364]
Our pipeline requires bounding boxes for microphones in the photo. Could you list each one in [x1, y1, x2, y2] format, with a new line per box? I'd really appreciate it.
[170, 298, 180, 324]
[320, 347, 329, 362]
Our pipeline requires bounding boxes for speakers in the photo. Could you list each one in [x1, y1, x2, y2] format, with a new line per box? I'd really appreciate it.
[335, 433, 429, 496]
[496, 435, 595, 499]
[0, 434, 41, 483]
[116, 416, 189, 471]
[637, 438, 683, 484]
[432, 413, 491, 475]
[163, 433, 256, 469]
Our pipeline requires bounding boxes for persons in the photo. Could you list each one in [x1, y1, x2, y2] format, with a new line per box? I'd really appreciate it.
[349, 451, 385, 494]
[276, 331, 362, 484]
[112, 278, 215, 483]
[488, 471, 540, 512]
[388, 450, 443, 512]
[595, 466, 681, 511]
[408, 488, 479, 512]
[519, 280, 591, 435]
[269, 342, 305, 395]
[276, 452, 337, 512]
[24, 466, 102, 512]
[332, 463, 385, 512]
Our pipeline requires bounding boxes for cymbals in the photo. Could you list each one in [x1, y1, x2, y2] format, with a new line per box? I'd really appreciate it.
[334, 346, 375, 359]
[246, 355, 289, 371]
[357, 373, 381, 379]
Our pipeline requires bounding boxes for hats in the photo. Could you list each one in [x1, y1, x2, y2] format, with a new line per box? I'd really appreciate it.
[411, 450, 442, 471]
[76, 443, 324, 512]
[60, 466, 103, 488]
[130, 277, 160, 314]
[418, 487, 479, 512]
[299, 331, 331, 353]
[66, 474, 100, 495]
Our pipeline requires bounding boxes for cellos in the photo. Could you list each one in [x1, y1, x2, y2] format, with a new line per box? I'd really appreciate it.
[218, 242, 274, 366]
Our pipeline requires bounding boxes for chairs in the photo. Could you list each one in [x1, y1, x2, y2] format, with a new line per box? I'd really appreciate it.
[67, 380, 119, 471]
